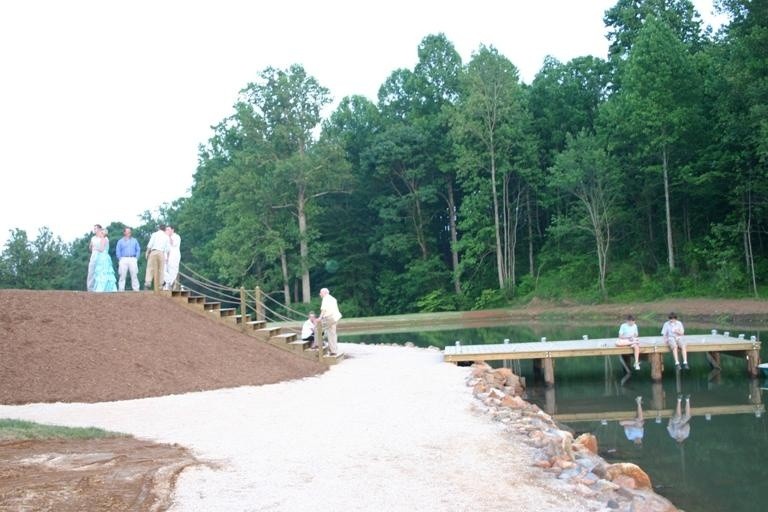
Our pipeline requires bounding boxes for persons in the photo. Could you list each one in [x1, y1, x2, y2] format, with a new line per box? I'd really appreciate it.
[618, 394, 647, 451]
[659, 312, 693, 371]
[313, 287, 344, 355]
[301, 310, 330, 351]
[162, 225, 182, 291]
[86, 223, 104, 292]
[614, 315, 643, 371]
[144, 224, 171, 291]
[91, 228, 119, 293]
[663, 392, 695, 448]
[115, 226, 142, 292]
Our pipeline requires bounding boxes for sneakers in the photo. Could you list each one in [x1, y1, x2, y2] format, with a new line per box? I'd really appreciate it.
[675, 363, 683, 371]
[635, 396, 643, 403]
[633, 362, 642, 371]
[676, 392, 685, 400]
[684, 393, 692, 399]
[681, 364, 691, 371]
